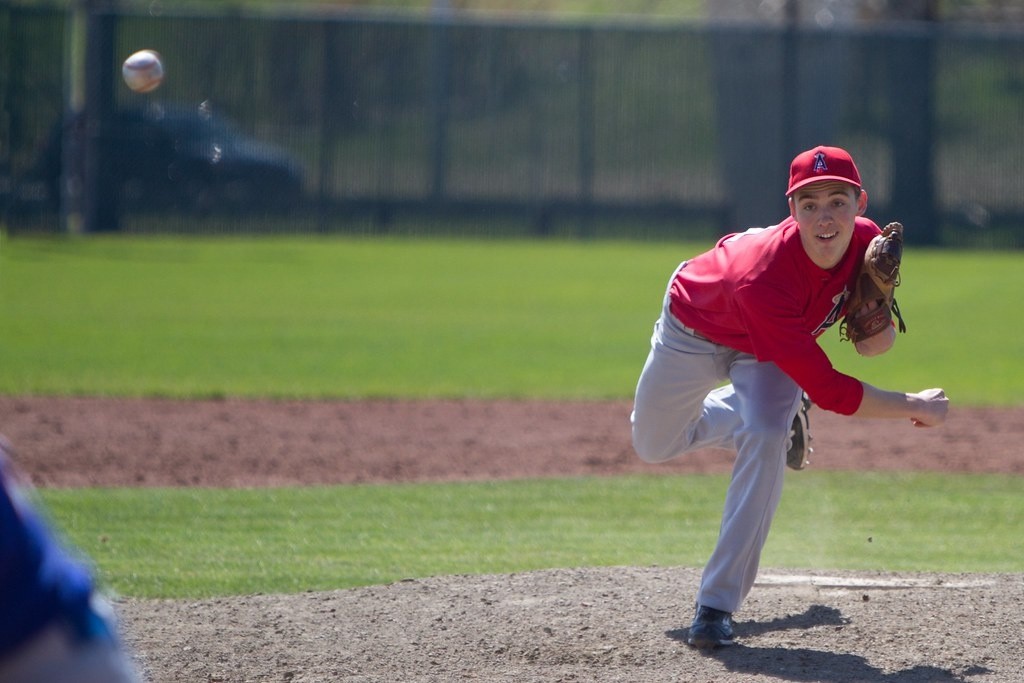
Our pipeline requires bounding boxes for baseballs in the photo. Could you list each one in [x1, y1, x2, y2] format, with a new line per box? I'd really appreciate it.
[121, 52, 165, 94]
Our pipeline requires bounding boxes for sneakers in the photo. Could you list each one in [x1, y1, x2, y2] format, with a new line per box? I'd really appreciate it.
[785, 388, 813, 471]
[688, 601, 734, 646]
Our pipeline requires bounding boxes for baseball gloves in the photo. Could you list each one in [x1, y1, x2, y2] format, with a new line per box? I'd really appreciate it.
[845, 219, 906, 342]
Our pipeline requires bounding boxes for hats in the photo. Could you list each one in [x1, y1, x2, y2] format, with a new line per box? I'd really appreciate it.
[785, 145, 861, 195]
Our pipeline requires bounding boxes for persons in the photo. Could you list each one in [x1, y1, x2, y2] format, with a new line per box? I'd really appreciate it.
[0, 441, 140, 683]
[629, 145, 950, 646]
